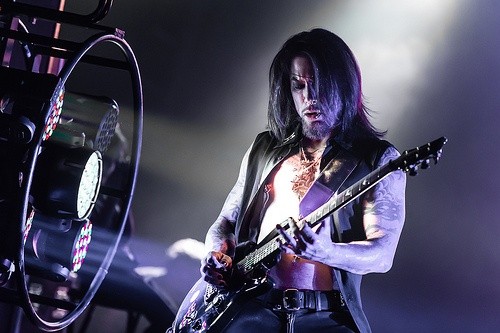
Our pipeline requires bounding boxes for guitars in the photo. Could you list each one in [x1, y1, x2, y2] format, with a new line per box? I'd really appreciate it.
[164, 136, 449, 333]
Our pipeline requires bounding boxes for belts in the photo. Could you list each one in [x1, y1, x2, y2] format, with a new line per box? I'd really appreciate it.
[254, 287, 349, 311]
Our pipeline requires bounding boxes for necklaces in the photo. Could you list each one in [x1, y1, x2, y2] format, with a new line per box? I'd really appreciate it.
[299, 145, 325, 174]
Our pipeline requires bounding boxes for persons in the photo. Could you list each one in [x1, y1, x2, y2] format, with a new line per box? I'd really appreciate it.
[201, 29, 406, 333]
